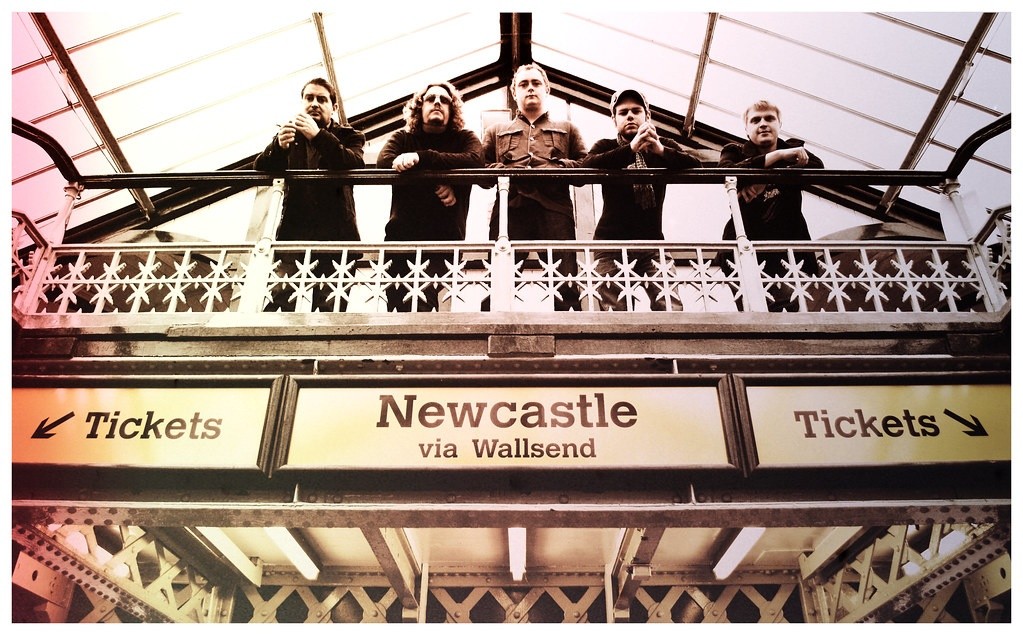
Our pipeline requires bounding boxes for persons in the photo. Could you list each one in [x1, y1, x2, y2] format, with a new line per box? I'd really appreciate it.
[580, 89, 702, 312]
[376, 81, 486, 311]
[480, 62, 586, 311]
[714, 100, 825, 313]
[255, 78, 366, 313]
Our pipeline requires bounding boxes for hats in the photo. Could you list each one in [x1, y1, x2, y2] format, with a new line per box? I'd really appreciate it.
[610, 87, 650, 117]
